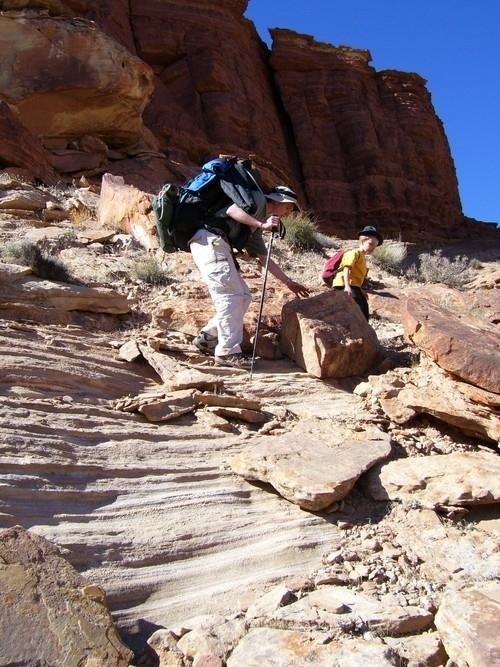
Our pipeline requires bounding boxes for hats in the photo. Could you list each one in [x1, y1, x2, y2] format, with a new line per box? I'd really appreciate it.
[263, 185, 301, 213]
[357, 225, 383, 247]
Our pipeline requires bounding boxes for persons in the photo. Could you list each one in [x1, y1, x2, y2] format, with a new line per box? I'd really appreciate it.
[331, 226, 383, 323]
[187, 186, 310, 370]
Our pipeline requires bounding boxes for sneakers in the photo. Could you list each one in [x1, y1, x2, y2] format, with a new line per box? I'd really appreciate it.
[214, 350, 256, 370]
[191, 331, 218, 356]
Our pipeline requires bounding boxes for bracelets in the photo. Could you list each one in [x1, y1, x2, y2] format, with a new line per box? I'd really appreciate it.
[285, 278, 291, 285]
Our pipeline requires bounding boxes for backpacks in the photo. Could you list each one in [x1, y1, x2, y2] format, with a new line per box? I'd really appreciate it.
[321, 245, 361, 287]
[151, 152, 266, 254]
